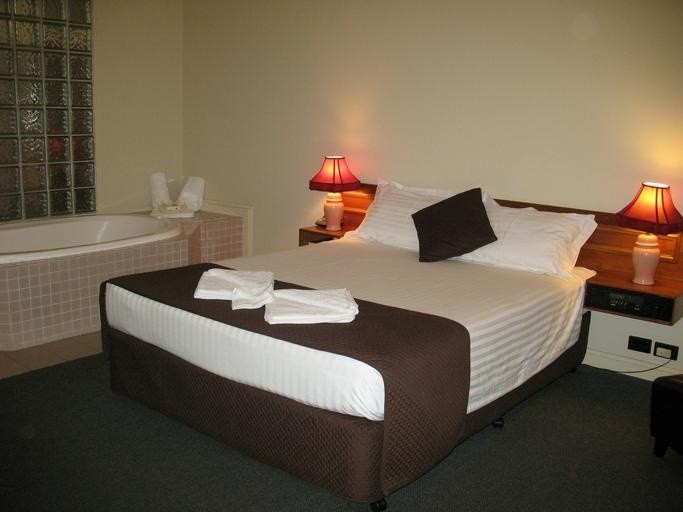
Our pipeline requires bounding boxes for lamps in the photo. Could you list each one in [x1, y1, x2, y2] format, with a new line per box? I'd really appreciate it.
[616, 182, 683, 287]
[309, 156, 360, 231]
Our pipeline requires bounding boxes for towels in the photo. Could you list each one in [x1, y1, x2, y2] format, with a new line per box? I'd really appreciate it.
[149, 172, 205, 218]
[193, 267, 359, 324]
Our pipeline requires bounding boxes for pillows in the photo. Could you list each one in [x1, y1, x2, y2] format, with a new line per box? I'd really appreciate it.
[341, 177, 599, 279]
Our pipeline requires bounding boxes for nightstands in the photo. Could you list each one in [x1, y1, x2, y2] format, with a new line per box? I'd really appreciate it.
[583, 272, 683, 325]
[299, 225, 356, 247]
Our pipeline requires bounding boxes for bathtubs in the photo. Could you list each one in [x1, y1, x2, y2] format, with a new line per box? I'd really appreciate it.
[0, 212, 181, 264]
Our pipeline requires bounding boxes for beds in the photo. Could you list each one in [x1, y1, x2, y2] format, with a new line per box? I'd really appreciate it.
[100, 238, 591, 512]
